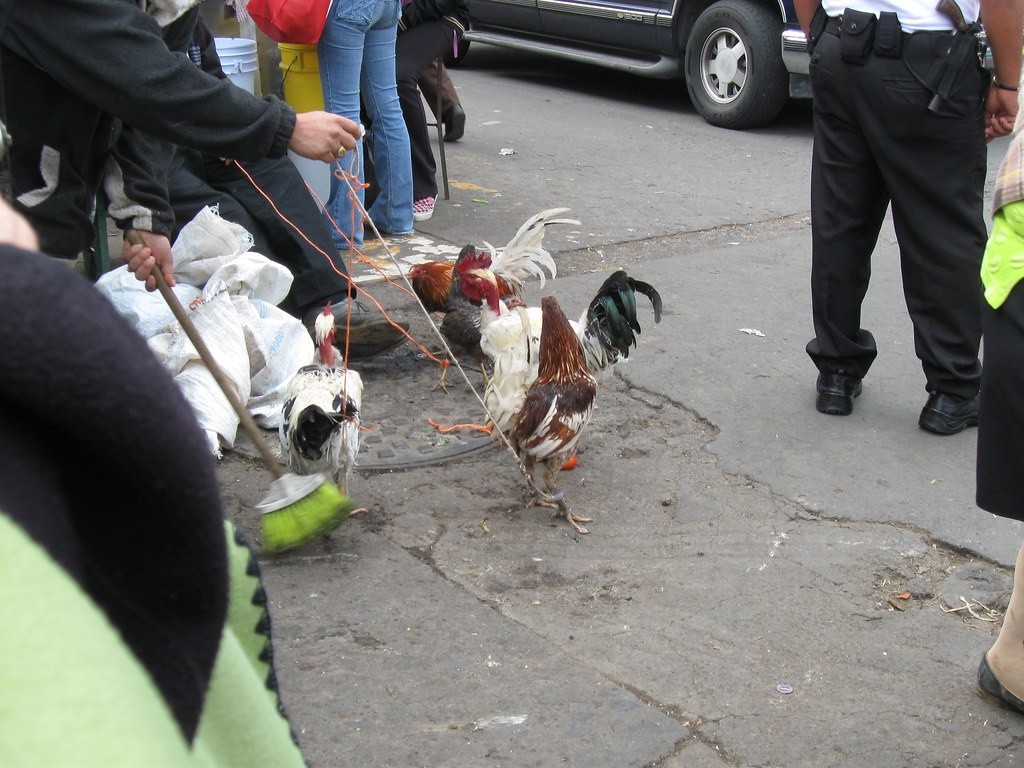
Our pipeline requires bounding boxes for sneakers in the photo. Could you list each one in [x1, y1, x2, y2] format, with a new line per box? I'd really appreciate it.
[302, 300, 412, 363]
[413, 193, 438, 221]
[816, 374, 863, 414]
[918, 388, 982, 433]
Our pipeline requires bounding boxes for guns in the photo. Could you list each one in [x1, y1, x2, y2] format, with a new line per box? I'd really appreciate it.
[926, 0, 984, 113]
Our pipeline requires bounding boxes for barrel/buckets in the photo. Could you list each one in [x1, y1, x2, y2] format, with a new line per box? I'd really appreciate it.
[213, 37, 258, 97]
[277, 42, 325, 114]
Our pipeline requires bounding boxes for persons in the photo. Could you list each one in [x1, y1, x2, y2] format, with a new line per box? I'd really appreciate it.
[0, 0, 472, 768]
[975, 82, 1024, 716]
[792, 0, 1024, 435]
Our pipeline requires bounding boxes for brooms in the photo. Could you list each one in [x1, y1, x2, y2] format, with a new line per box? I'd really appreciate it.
[123, 226, 354, 554]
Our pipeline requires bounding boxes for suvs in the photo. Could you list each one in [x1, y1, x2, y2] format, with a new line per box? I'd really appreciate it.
[440, 0, 997, 131]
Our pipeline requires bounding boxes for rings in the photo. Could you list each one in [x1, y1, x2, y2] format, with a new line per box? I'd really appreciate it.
[334, 145, 346, 157]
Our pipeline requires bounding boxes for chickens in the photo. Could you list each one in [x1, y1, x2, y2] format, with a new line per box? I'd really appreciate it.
[278, 299, 364, 497]
[512, 295, 600, 535]
[427, 242, 528, 396]
[451, 250, 664, 449]
[409, 205, 583, 314]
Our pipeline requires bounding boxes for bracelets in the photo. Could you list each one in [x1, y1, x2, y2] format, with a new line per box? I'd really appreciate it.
[992, 80, 1020, 91]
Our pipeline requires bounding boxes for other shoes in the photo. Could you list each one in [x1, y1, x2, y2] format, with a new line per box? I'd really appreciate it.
[976, 651, 1024, 711]
[443, 104, 466, 142]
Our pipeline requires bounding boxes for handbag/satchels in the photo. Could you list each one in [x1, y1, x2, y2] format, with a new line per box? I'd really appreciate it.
[245, 0, 331, 46]
